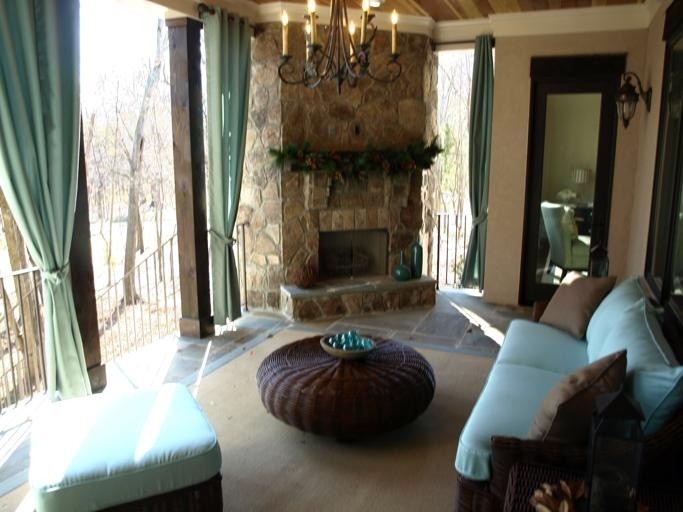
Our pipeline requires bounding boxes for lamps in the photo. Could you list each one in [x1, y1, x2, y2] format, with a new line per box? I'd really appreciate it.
[616, 71, 652, 131]
[276, 0, 406, 95]
[572, 167, 591, 204]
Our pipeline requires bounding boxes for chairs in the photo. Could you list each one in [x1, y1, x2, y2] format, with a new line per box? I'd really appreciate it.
[541, 199, 590, 282]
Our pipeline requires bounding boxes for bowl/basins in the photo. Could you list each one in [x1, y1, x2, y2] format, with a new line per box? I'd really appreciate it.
[320, 332, 376, 361]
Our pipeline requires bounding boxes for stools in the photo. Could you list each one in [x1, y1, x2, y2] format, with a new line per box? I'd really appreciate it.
[30, 382, 223, 512]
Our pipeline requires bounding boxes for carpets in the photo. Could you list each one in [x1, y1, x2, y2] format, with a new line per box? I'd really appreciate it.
[0, 328, 499, 511]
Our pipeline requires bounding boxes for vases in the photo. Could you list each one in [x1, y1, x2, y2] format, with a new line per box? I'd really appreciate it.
[393, 247, 412, 281]
[412, 240, 422, 279]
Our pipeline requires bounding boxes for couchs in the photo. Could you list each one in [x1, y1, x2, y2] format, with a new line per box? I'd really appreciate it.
[454, 272, 682, 512]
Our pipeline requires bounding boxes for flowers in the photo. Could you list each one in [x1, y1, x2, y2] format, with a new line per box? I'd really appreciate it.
[267, 133, 445, 177]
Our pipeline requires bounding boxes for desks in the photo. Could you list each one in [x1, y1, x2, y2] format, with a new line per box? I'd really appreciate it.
[504, 463, 665, 511]
[574, 207, 592, 235]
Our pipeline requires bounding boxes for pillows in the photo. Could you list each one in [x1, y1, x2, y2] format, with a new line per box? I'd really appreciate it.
[587, 298, 682, 436]
[562, 205, 578, 243]
[538, 270, 616, 339]
[524, 349, 628, 441]
[583, 274, 656, 348]
